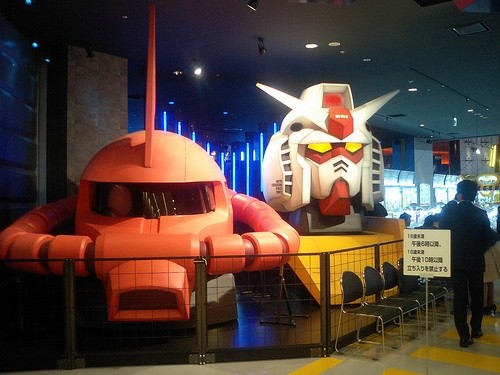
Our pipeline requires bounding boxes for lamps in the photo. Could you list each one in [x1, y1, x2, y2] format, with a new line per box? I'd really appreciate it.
[246, 0, 258, 12]
[86, 40, 95, 59]
[258, 38, 267, 55]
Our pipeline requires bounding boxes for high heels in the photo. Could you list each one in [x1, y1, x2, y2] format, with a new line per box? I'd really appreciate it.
[484, 304, 497, 314]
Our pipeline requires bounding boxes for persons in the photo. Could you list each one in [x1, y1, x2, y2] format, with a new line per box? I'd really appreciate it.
[439, 179, 493, 348]
[432, 213, 440, 228]
[483, 228, 500, 315]
[255, 83, 404, 304]
[414, 214, 437, 229]
[0, 0, 301, 333]
[399, 212, 411, 226]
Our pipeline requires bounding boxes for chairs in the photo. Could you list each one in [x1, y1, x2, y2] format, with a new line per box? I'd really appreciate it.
[334, 258, 456, 361]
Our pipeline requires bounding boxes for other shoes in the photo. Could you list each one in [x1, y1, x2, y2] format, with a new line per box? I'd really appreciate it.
[460, 338, 473, 347]
[471, 331, 483, 338]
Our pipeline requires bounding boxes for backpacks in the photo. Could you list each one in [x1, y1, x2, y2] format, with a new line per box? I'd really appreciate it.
[397, 260, 418, 293]
